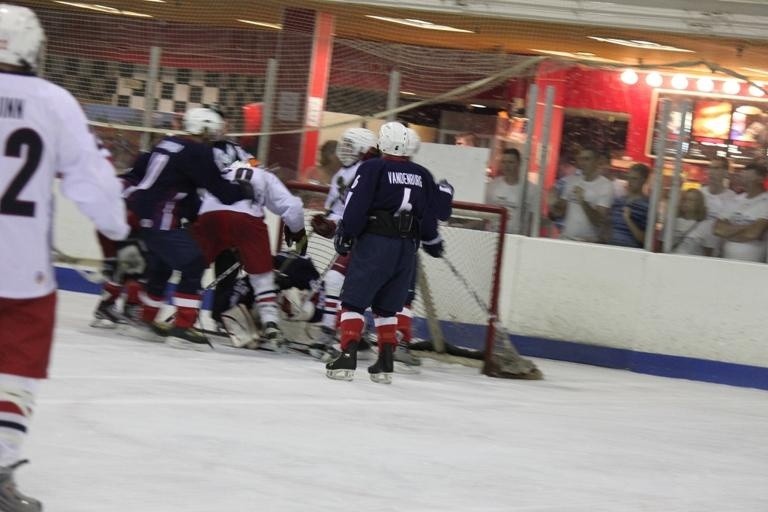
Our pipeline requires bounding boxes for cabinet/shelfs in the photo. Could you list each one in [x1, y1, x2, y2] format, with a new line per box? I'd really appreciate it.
[644, 87, 768, 171]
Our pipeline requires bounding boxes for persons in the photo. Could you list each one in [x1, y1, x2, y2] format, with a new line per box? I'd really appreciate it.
[710, 163, 768, 263]
[544, 146, 617, 245]
[595, 163, 651, 250]
[697, 156, 741, 216]
[658, 188, 725, 261]
[482, 147, 537, 238]
[90, 105, 455, 373]
[0, 4, 135, 511]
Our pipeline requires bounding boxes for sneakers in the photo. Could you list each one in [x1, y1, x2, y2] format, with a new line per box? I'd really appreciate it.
[96, 302, 283, 344]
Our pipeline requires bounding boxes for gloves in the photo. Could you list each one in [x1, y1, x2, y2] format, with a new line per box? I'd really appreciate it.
[113, 229, 149, 279]
[438, 179, 454, 200]
[311, 213, 336, 239]
[284, 225, 309, 256]
[233, 177, 256, 200]
[332, 220, 351, 255]
[423, 234, 445, 258]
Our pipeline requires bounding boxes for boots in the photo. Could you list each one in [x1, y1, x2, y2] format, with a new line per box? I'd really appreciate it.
[0, 458, 41, 511]
[310, 331, 422, 374]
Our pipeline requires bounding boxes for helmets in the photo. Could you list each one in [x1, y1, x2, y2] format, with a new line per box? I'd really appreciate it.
[184, 108, 228, 137]
[335, 127, 379, 167]
[0, 5, 47, 70]
[378, 122, 421, 158]
